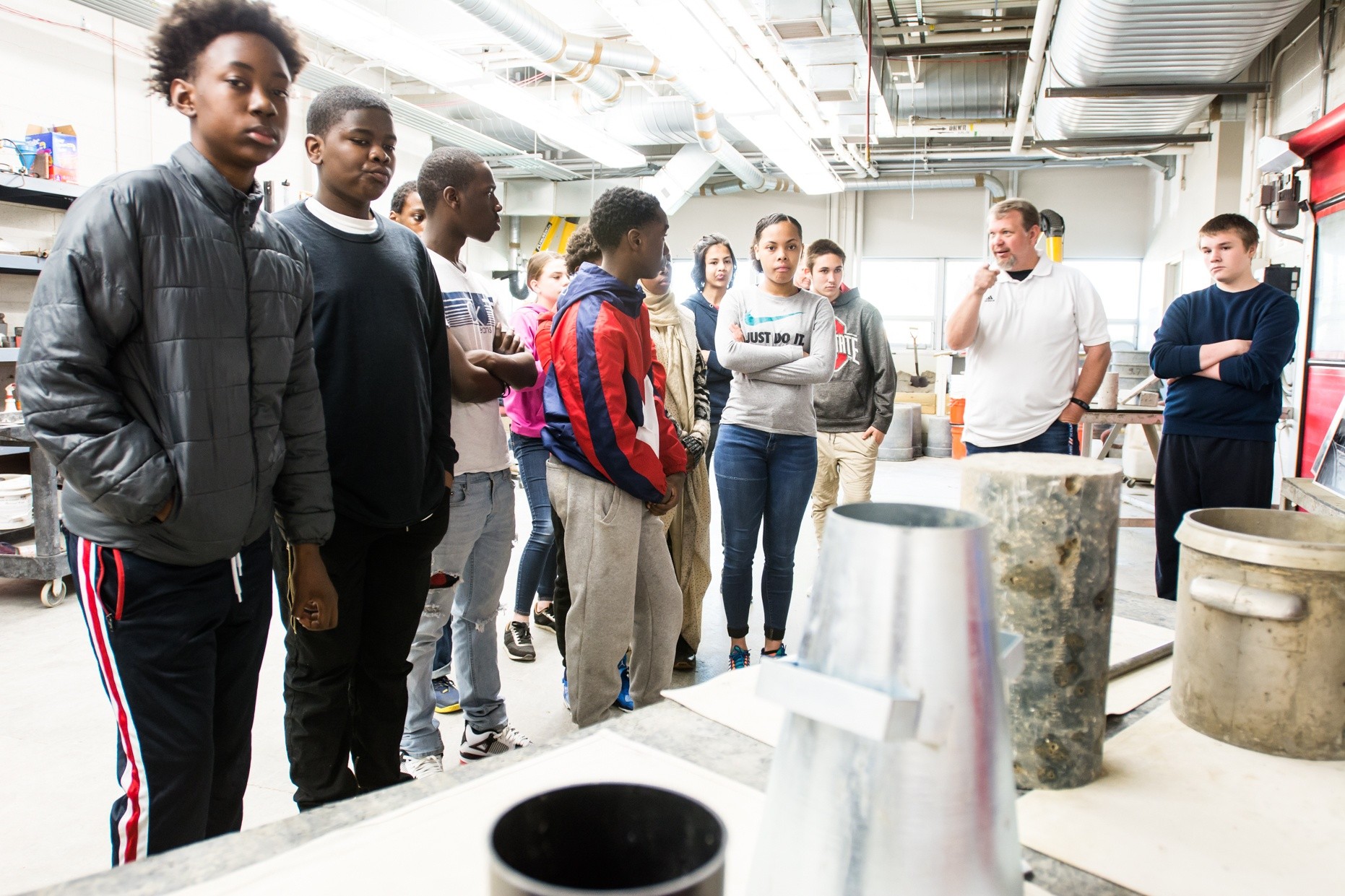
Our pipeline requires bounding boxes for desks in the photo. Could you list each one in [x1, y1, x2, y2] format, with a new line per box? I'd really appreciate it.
[12, 582, 1345, 896]
[1081, 404, 1165, 528]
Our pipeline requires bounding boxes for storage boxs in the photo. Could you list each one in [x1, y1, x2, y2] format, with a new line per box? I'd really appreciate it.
[25, 124, 78, 182]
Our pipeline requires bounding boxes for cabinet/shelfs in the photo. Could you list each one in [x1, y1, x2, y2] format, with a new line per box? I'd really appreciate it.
[0, 173, 88, 455]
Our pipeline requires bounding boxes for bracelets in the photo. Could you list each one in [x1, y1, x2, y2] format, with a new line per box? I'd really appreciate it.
[1071, 397, 1090, 411]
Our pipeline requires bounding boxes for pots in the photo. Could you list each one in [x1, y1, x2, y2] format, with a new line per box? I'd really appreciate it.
[1169, 507, 1345, 764]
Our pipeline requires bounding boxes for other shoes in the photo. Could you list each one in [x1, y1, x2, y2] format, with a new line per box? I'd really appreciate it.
[674, 636, 697, 671]
[562, 670, 570, 710]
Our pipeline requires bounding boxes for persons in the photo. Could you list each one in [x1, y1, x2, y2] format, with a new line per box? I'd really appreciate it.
[946, 200, 1112, 455]
[387, 144, 898, 780]
[15, 0, 339, 871]
[261, 82, 459, 815]
[1148, 212, 1301, 600]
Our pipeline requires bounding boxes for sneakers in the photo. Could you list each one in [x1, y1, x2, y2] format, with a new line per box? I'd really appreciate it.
[505, 621, 537, 661]
[760, 643, 787, 664]
[432, 675, 463, 714]
[731, 644, 750, 672]
[534, 603, 554, 632]
[405, 755, 443, 779]
[614, 655, 634, 711]
[459, 720, 529, 766]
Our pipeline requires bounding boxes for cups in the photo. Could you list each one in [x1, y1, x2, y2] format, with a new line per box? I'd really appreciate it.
[486, 781, 729, 896]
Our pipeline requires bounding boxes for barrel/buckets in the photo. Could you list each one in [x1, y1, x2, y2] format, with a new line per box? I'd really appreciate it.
[923, 414, 952, 459]
[949, 372, 966, 459]
[0, 474, 33, 531]
[876, 401, 923, 461]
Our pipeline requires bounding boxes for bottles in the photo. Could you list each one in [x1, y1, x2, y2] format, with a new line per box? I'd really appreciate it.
[0, 313, 23, 348]
